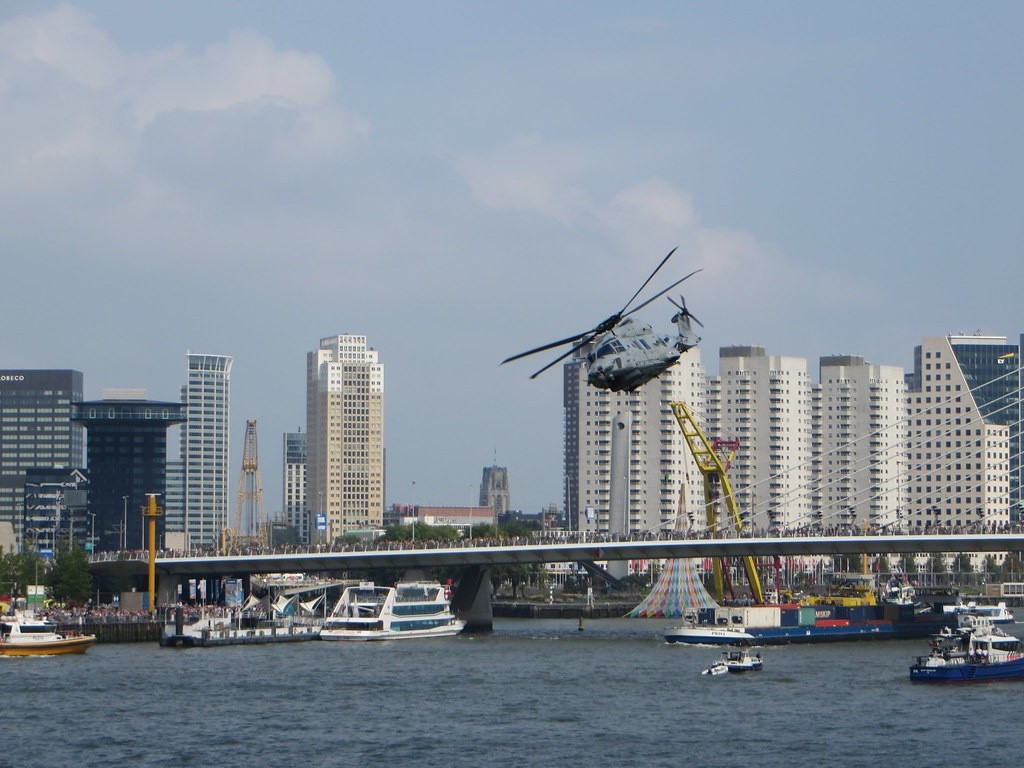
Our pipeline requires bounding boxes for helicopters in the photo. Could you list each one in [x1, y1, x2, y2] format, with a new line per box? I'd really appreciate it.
[499, 243, 705, 393]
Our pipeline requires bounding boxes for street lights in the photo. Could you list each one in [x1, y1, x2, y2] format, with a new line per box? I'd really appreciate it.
[90, 513, 96, 560]
[566, 475, 572, 532]
[16, 502, 22, 551]
[467, 484, 472, 539]
[123, 495, 129, 550]
[140, 506, 147, 550]
[317, 491, 325, 544]
[411, 480, 417, 541]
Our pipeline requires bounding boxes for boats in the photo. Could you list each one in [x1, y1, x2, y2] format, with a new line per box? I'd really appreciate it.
[712, 651, 764, 671]
[319, 581, 467, 641]
[0, 619, 97, 657]
[909, 625, 1023, 681]
[961, 615, 995, 631]
[663, 585, 959, 645]
[702, 666, 729, 676]
[917, 601, 1014, 623]
